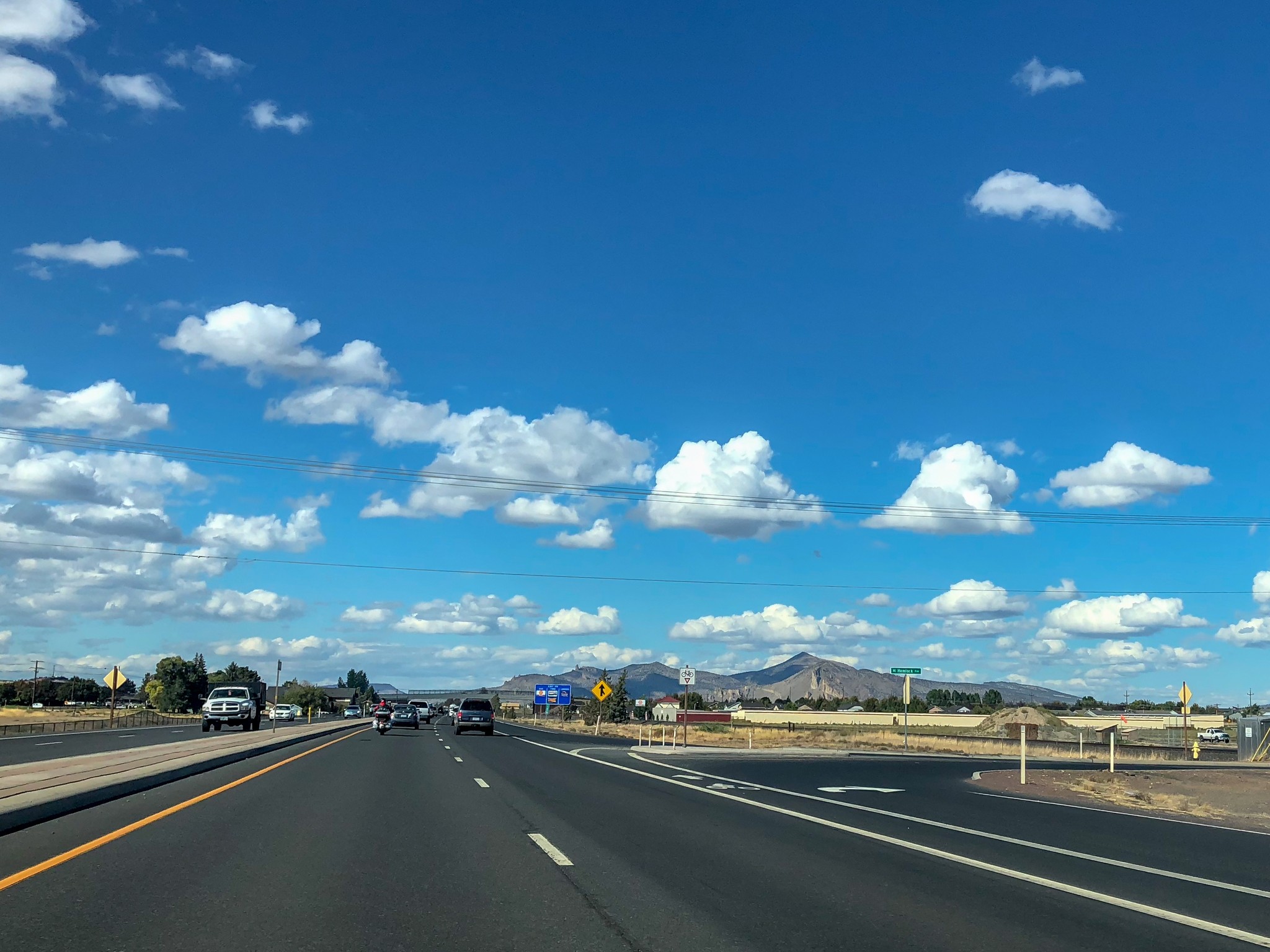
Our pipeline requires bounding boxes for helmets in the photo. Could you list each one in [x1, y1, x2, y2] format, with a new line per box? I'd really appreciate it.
[380, 698, 385, 703]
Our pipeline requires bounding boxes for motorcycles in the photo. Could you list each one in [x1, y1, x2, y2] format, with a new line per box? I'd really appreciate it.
[375, 710, 390, 735]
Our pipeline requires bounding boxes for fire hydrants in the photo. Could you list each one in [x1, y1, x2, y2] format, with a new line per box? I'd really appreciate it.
[1191, 742, 1201, 760]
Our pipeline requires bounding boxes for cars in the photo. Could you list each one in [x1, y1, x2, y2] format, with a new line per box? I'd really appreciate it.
[269, 703, 295, 721]
[429, 703, 459, 719]
[343, 705, 362, 718]
[368, 704, 380, 712]
[64, 700, 134, 710]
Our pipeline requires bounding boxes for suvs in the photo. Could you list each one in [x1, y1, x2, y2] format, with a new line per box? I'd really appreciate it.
[390, 704, 422, 730]
[201, 686, 261, 731]
[453, 698, 495, 736]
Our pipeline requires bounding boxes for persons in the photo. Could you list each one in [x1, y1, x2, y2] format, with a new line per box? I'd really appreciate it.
[373, 697, 394, 731]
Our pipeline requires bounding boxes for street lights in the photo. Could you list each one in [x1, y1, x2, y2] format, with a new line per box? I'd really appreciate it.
[1124, 690, 1130, 704]
[1247, 688, 1254, 707]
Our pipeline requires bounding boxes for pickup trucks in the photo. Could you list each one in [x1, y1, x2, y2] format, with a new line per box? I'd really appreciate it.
[408, 700, 430, 724]
[1197, 728, 1229, 743]
